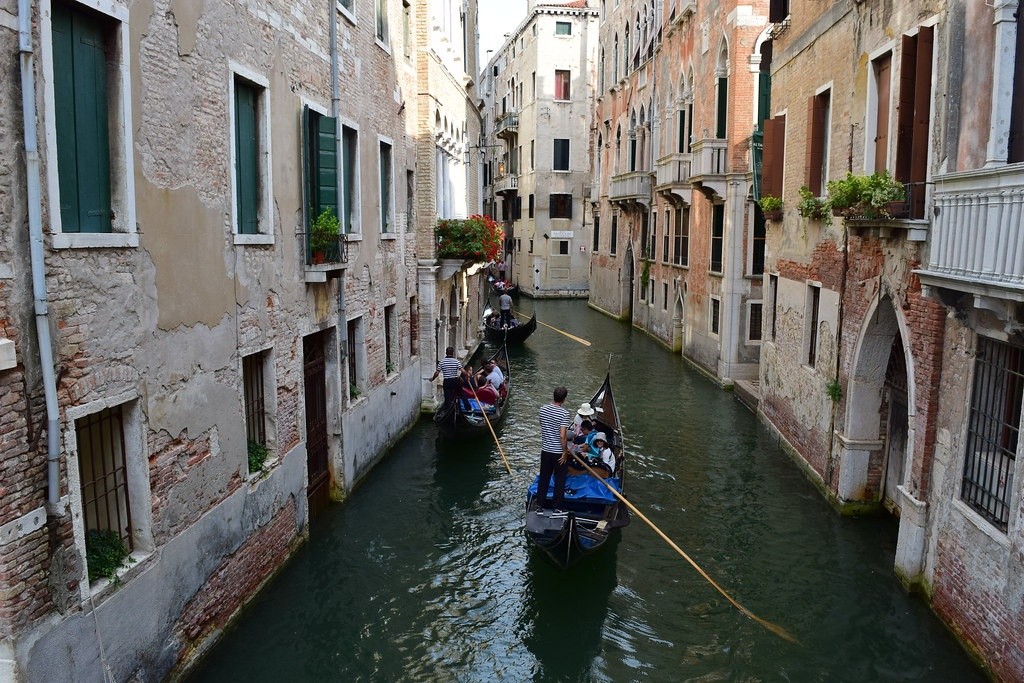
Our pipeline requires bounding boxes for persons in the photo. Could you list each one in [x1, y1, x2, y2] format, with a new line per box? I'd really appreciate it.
[499, 260, 508, 279]
[534, 387, 570, 515]
[567, 403, 616, 473]
[494, 278, 515, 291]
[499, 290, 513, 330]
[429, 347, 473, 412]
[487, 308, 518, 328]
[459, 359, 504, 405]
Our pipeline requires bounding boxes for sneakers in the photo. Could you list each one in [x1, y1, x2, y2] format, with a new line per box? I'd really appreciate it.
[534, 507, 544, 515]
[551, 508, 570, 516]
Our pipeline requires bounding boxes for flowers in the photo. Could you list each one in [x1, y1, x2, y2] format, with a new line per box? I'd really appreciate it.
[434, 213, 506, 264]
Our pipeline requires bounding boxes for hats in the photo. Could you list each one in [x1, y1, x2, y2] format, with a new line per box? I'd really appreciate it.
[576, 402, 596, 416]
[594, 432, 608, 443]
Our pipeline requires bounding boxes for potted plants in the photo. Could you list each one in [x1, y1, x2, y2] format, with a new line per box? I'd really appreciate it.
[797, 169, 906, 240]
[309, 205, 342, 263]
[758, 193, 783, 220]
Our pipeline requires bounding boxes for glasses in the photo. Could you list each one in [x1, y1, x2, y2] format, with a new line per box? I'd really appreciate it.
[595, 439, 605, 442]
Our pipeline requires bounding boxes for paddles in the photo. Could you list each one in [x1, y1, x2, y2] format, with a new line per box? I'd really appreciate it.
[468, 378, 514, 476]
[567, 446, 744, 611]
[513, 310, 592, 346]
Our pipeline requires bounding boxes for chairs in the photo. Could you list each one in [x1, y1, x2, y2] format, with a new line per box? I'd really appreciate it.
[478, 388, 496, 404]
[615, 449, 623, 465]
[501, 382, 506, 398]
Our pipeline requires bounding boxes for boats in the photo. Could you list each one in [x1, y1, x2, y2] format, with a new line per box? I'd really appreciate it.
[481, 296, 537, 345]
[487, 268, 519, 298]
[431, 324, 510, 445]
[523, 353, 631, 572]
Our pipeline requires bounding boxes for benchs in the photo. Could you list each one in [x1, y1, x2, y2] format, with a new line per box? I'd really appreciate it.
[565, 453, 613, 479]
